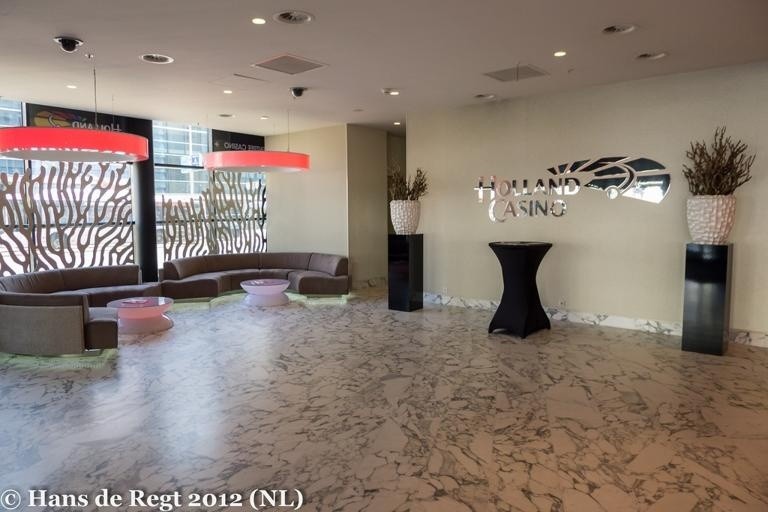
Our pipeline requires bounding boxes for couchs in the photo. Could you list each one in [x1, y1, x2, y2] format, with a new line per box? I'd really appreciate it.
[161, 254, 348, 306]
[0, 264, 161, 357]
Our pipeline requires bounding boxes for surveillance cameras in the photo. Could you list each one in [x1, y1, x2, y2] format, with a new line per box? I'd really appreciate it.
[55, 37, 83, 51]
[288, 87, 306, 97]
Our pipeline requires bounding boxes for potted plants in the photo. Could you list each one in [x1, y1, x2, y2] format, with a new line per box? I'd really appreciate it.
[387, 159, 428, 236]
[680, 125, 757, 246]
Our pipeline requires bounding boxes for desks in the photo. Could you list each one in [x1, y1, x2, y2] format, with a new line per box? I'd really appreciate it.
[488, 241, 552, 338]
